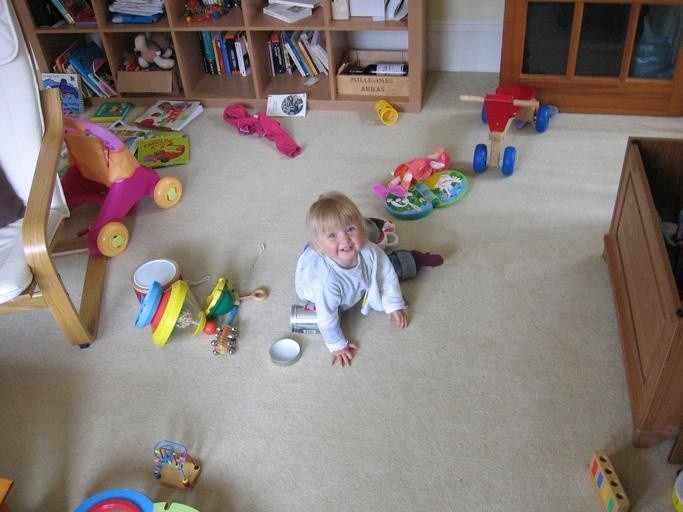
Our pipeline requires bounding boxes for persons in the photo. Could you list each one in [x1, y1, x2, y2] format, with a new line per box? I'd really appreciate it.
[295, 191, 445, 369]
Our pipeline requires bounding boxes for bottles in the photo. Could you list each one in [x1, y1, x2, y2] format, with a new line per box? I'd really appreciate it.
[348, 61, 409, 76]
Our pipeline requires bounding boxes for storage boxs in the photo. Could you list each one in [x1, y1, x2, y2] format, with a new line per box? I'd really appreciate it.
[336, 48, 410, 96]
[115, 55, 182, 96]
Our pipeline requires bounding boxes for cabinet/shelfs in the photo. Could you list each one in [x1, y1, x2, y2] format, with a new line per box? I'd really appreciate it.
[13, 0, 430, 113]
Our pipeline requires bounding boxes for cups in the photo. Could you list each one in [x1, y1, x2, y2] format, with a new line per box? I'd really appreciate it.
[373, 100, 399, 126]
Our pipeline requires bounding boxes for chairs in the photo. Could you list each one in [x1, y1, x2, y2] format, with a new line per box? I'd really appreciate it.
[1, 0, 107, 348]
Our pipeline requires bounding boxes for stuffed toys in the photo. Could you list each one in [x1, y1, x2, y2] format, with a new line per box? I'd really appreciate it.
[371, 146, 453, 199]
[134, 33, 176, 69]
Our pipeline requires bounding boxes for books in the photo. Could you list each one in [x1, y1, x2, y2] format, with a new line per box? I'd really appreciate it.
[267, 31, 330, 77]
[52, 0, 90, 25]
[94, 102, 131, 119]
[263, 1, 320, 24]
[53, 39, 118, 99]
[41, 73, 84, 113]
[195, 30, 253, 76]
[332, 1, 410, 23]
[134, 99, 204, 131]
